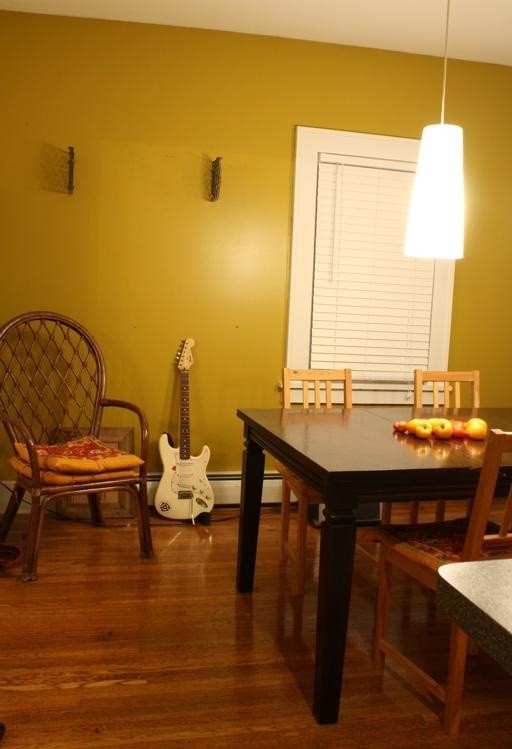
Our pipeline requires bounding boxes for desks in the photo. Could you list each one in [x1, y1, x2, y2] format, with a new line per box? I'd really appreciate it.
[428, 550, 511, 677]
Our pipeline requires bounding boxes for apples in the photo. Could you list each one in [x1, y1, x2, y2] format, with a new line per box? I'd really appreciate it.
[394, 418, 487, 439]
[393, 430, 488, 460]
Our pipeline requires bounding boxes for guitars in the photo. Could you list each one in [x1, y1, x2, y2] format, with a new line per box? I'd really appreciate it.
[154, 338, 215, 525]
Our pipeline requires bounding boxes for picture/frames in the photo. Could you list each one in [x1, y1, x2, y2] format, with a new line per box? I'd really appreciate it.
[47, 424, 139, 521]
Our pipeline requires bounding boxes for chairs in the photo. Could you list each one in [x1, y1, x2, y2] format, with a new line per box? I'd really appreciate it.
[407, 367, 481, 410]
[365, 423, 511, 739]
[272, 367, 393, 604]
[0, 309, 155, 585]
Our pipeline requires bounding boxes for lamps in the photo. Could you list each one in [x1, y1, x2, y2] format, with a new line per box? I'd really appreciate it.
[400, 1, 472, 263]
